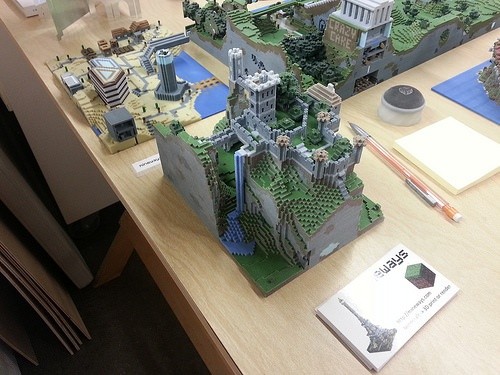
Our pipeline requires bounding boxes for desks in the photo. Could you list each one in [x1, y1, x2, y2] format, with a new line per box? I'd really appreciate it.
[1, 0, 499, 374]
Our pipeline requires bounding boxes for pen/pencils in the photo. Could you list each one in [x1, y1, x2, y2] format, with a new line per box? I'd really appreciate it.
[347, 119, 462, 221]
